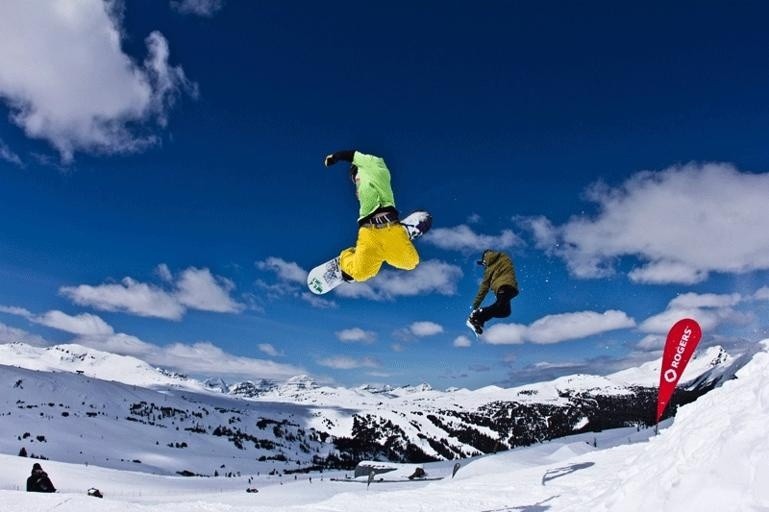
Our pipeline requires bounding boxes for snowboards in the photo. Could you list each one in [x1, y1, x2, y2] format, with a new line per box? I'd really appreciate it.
[307, 211, 432, 294]
[466, 308, 482, 336]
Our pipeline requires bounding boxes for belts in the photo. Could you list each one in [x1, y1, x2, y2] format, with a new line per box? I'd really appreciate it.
[364, 214, 397, 224]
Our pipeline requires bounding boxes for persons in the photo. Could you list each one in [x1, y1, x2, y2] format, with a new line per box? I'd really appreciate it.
[323, 149, 420, 283]
[469, 249, 521, 335]
[26, 463, 59, 493]
